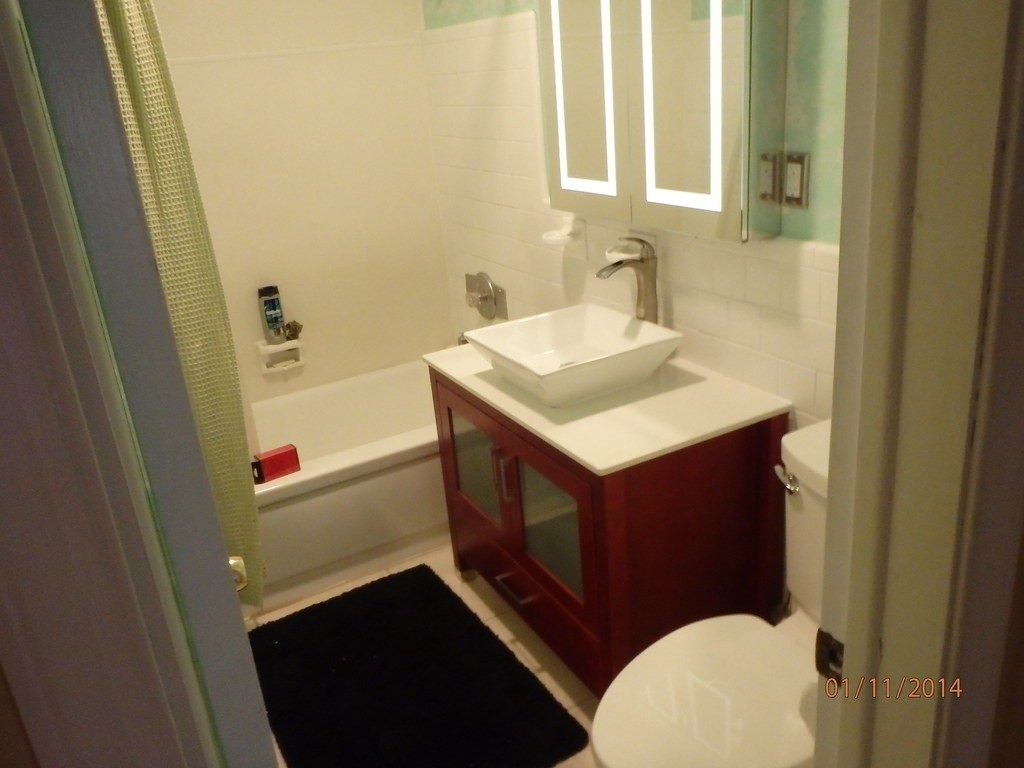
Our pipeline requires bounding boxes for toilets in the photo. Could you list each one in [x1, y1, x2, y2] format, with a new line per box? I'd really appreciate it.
[584, 417, 831, 767]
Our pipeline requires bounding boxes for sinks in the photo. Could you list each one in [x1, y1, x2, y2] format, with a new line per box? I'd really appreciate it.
[462, 301, 683, 409]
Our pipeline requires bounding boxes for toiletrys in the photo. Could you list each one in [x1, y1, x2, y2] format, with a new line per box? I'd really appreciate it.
[257, 285, 287, 347]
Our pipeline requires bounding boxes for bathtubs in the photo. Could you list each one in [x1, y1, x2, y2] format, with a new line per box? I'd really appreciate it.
[237, 359, 449, 624]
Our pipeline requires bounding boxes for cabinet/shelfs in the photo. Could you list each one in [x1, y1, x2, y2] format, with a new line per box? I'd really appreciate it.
[428, 376, 793, 702]
[538, 0, 787, 242]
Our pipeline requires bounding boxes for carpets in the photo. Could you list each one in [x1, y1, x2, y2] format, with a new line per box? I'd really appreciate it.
[243, 563, 592, 766]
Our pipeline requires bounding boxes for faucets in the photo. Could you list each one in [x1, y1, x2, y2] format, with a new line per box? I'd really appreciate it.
[595, 237, 660, 327]
